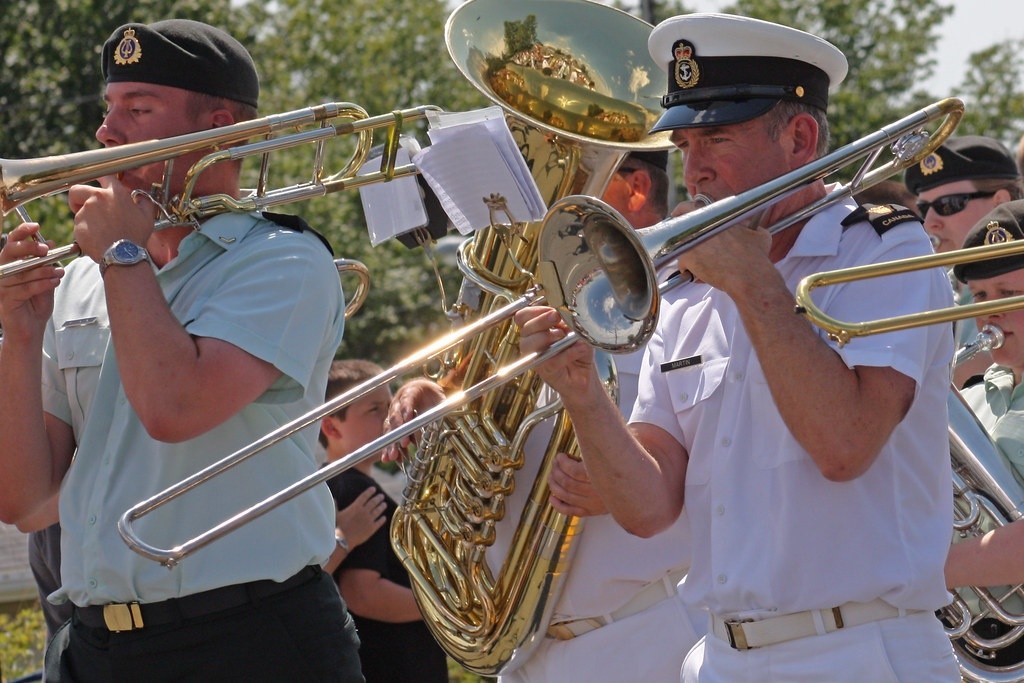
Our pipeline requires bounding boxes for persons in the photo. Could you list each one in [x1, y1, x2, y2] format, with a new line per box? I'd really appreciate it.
[323, 486, 387, 574]
[0, 18, 366, 683]
[382, 149, 699, 683]
[515, 11, 965, 683]
[317, 359, 448, 683]
[851, 135, 1024, 683]
[28, 523, 74, 649]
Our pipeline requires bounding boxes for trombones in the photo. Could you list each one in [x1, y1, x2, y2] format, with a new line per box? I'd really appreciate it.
[117, 95, 967, 570]
[0, 99, 445, 277]
[791, 239, 1024, 350]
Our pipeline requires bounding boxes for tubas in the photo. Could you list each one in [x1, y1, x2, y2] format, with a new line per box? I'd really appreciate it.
[932, 325, 1024, 683]
[388, 1, 683, 676]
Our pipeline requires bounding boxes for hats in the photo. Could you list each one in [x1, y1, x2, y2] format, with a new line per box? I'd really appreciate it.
[901, 136, 1019, 194]
[952, 198, 1024, 284]
[627, 150, 670, 172]
[101, 19, 260, 108]
[646, 13, 849, 135]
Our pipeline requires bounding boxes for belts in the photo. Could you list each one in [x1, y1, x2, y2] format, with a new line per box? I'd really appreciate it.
[543, 575, 679, 642]
[709, 600, 929, 654]
[73, 560, 323, 634]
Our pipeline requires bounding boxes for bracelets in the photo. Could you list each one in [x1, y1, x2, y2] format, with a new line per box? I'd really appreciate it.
[336, 536, 348, 549]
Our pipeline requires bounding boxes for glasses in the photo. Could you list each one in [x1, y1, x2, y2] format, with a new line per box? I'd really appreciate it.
[915, 190, 995, 220]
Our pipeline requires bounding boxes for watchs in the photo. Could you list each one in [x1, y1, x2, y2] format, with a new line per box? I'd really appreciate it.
[99, 239, 152, 279]
